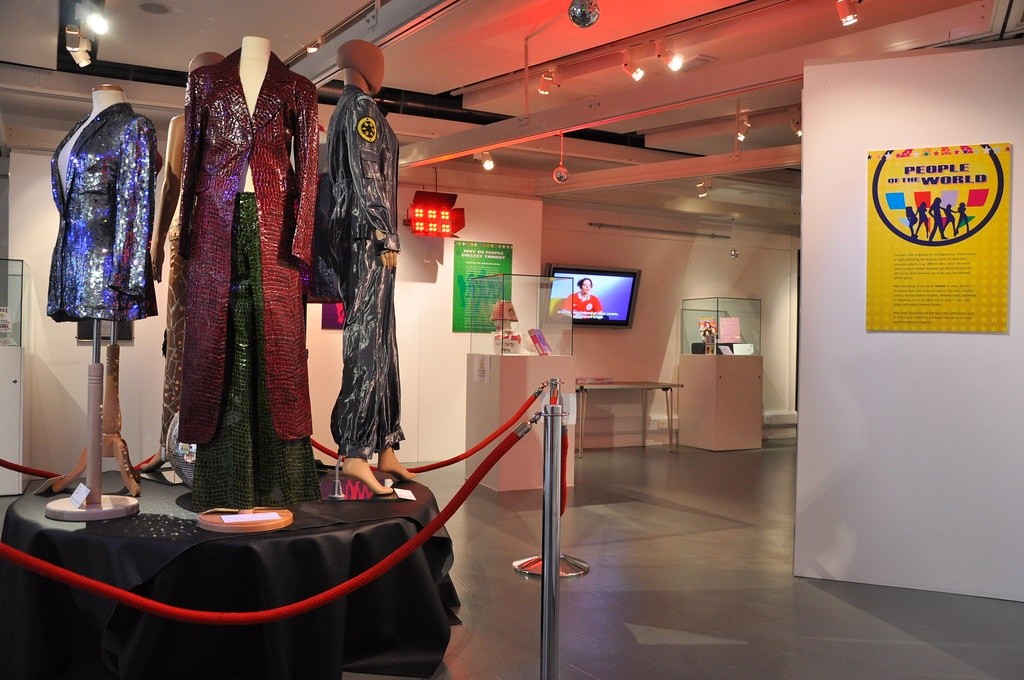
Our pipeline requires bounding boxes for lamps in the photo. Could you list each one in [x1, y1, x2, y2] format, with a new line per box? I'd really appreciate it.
[697, 182, 707, 197]
[622, 53, 644, 81]
[481, 152, 494, 171]
[656, 42, 683, 71]
[788, 120, 802, 137]
[835, 0, 857, 26]
[538, 71, 553, 95]
[738, 118, 752, 141]
[66, 24, 80, 51]
[728, 218, 739, 259]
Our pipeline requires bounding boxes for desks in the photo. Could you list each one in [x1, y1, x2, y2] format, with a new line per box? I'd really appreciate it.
[0, 462, 462, 680]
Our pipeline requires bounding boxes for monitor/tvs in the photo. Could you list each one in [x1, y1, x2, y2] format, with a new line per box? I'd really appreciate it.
[542, 263, 643, 329]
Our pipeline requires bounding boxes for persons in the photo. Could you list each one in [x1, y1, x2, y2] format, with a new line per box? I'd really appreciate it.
[701, 319, 717, 342]
[565, 278, 602, 316]
[146, 36, 318, 513]
[328, 38, 418, 496]
[49, 86, 159, 321]
